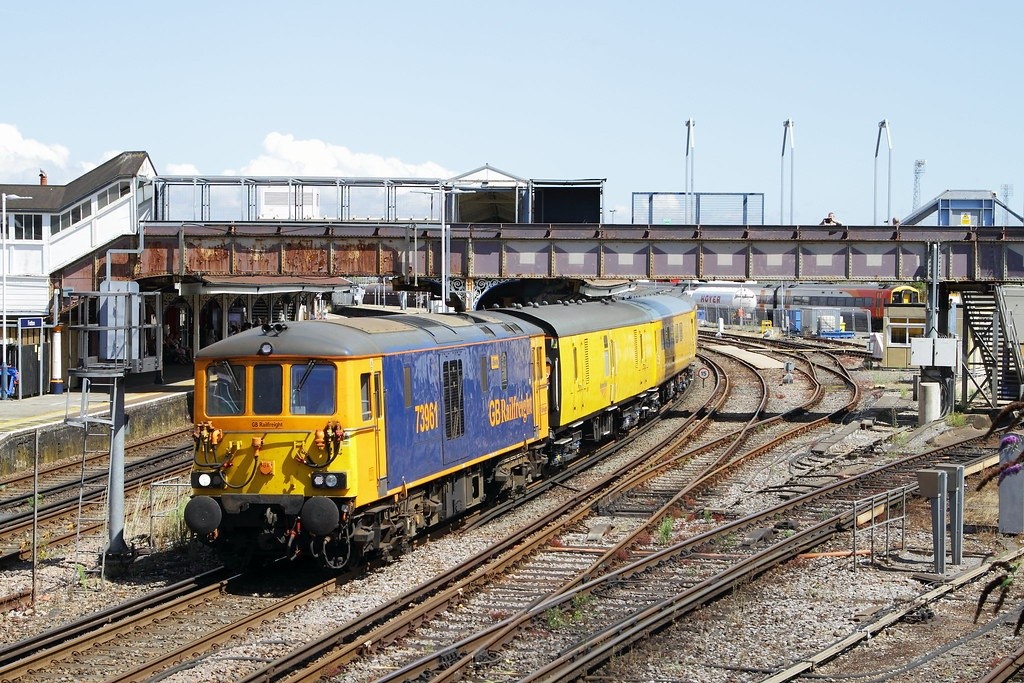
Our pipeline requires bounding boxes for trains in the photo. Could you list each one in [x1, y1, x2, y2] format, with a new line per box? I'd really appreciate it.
[182, 278, 701, 581]
[692, 281, 920, 338]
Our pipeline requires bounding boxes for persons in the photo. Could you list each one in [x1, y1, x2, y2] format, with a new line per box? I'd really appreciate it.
[166, 318, 261, 363]
